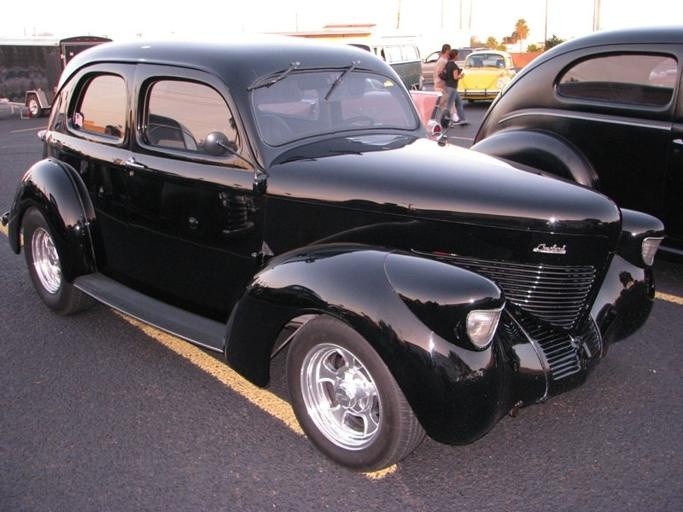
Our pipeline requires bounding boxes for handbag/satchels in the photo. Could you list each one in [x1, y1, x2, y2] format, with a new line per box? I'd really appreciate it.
[439, 70, 449, 80]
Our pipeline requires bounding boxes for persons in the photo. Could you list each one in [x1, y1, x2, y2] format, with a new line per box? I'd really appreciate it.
[428, 44, 459, 126]
[445, 50, 471, 128]
